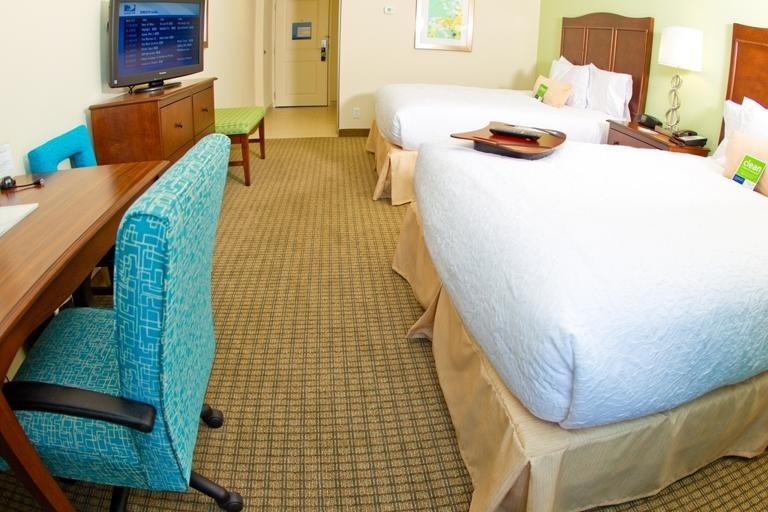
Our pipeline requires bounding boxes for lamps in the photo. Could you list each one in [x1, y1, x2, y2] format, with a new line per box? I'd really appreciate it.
[655, 21, 705, 138]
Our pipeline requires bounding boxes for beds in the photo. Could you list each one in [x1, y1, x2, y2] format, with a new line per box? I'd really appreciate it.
[365, 11, 654, 207]
[392, 22, 768, 512]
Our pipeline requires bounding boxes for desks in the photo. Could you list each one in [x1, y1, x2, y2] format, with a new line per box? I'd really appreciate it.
[1, 160, 172, 511]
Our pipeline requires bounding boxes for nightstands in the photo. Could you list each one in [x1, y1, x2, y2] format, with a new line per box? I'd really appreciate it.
[606, 119, 711, 157]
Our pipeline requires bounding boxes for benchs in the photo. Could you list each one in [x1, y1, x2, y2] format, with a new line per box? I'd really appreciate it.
[212, 106, 267, 186]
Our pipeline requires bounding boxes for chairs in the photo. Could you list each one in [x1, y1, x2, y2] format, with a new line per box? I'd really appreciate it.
[27, 125, 114, 295]
[0, 133, 243, 511]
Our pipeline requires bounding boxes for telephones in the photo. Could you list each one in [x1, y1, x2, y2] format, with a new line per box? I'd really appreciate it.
[669, 129, 708, 147]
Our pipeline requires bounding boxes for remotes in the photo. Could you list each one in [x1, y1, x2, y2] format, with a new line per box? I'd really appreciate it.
[490, 127, 541, 141]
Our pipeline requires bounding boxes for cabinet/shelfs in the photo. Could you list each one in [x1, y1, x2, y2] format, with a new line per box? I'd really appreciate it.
[89, 76, 218, 165]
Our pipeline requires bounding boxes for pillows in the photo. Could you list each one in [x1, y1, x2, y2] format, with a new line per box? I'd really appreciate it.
[532, 56, 634, 120]
[705, 96, 768, 197]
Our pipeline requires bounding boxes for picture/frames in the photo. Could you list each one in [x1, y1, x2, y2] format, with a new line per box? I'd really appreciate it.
[414, 1, 473, 53]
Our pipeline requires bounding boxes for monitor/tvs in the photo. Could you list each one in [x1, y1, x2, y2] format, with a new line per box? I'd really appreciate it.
[107, 1, 205, 92]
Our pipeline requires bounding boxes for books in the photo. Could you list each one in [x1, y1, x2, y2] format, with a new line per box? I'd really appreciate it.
[732, 154, 767, 191]
[534, 83, 548, 102]
